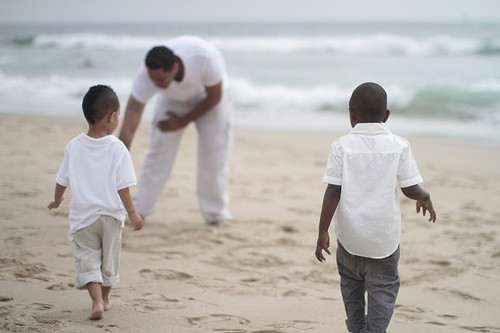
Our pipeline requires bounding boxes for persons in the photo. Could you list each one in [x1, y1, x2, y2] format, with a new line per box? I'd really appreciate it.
[119, 36, 233, 229]
[315, 82, 437, 333]
[47, 84, 143, 320]
